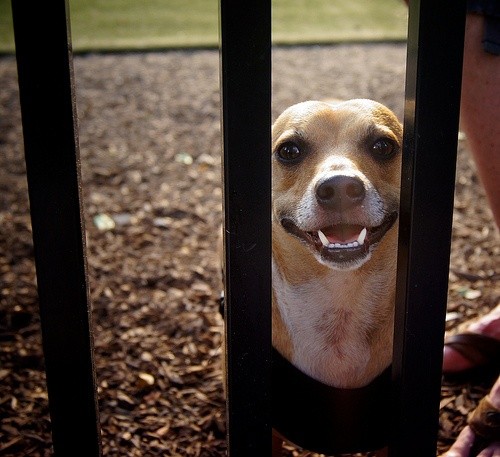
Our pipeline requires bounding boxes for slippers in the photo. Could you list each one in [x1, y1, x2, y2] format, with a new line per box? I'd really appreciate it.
[440, 396, 500, 456]
[443, 318, 500, 380]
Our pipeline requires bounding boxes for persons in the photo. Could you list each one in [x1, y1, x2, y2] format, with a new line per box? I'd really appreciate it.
[440, 0, 500, 457]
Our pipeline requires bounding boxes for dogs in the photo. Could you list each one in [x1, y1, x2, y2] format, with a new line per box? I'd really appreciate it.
[271, 97, 404, 457]
